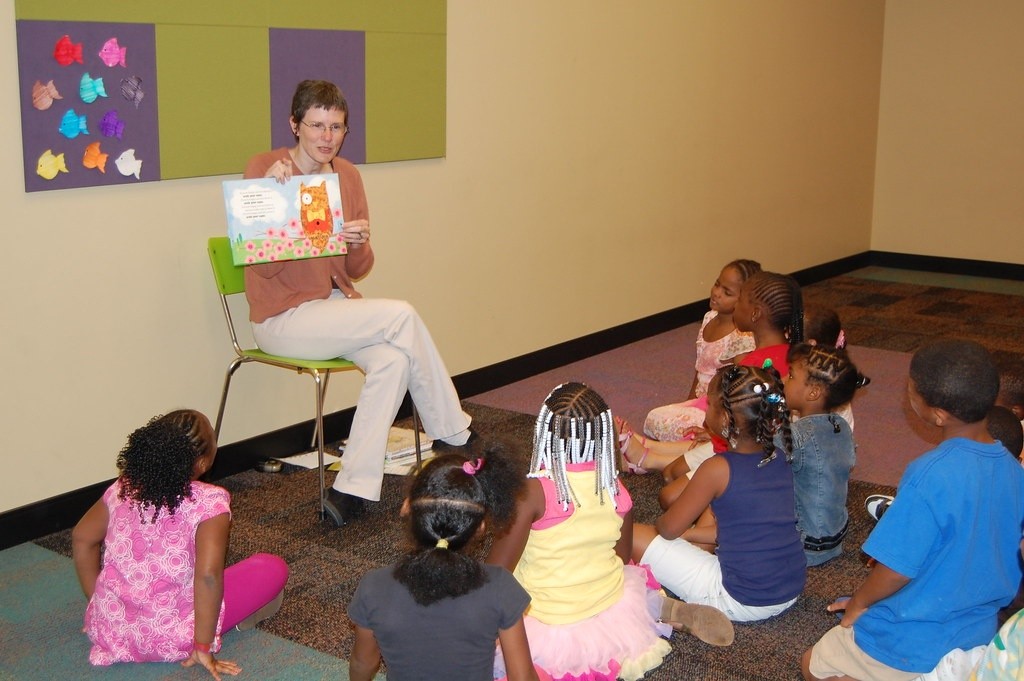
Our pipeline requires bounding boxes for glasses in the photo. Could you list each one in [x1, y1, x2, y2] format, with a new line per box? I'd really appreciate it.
[299, 121, 350, 135]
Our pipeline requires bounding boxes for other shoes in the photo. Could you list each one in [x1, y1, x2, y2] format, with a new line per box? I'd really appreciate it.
[236, 589, 285, 632]
[661, 597, 734, 647]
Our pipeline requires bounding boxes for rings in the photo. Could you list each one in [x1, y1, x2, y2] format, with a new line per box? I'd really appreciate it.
[359, 232, 368, 240]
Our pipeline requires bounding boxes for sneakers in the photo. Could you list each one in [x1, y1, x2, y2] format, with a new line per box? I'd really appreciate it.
[431, 429, 483, 459]
[864, 494, 894, 523]
[322, 487, 351, 527]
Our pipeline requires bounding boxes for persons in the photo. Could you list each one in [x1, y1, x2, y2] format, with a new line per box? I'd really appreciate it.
[487, 381, 735, 681]
[346, 446, 539, 681]
[71, 408, 288, 681]
[243, 80, 491, 526]
[901, 607, 1024, 681]
[610, 259, 870, 622]
[801, 338, 1024, 681]
[864, 407, 1024, 568]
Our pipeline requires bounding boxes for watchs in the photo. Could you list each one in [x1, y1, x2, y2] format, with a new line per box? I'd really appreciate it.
[192, 640, 216, 653]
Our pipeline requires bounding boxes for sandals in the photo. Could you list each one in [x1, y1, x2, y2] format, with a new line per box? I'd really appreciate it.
[614, 415, 645, 446]
[617, 420, 649, 475]
